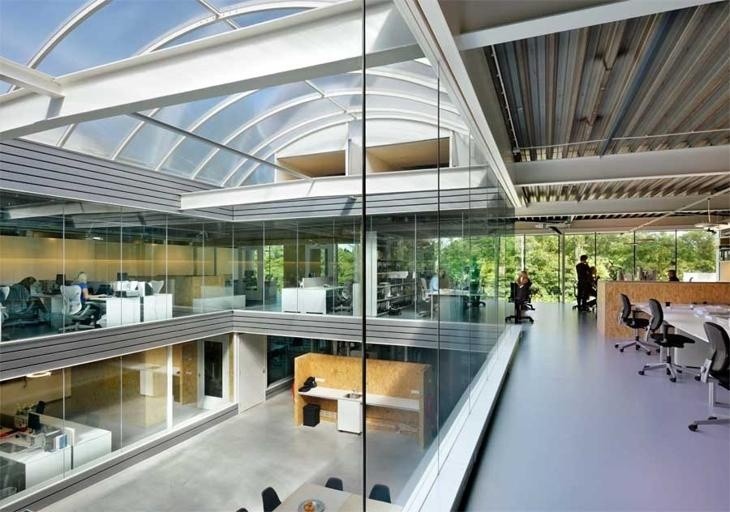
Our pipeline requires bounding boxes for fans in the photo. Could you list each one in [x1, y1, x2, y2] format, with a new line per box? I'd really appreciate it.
[694, 198, 721, 232]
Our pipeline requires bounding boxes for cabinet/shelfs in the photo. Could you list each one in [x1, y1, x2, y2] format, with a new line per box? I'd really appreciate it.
[377, 233, 414, 317]
[715, 216, 730, 284]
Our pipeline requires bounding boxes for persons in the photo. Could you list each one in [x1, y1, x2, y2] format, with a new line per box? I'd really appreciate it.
[576, 255, 591, 311]
[429, 269, 445, 312]
[515, 270, 531, 303]
[667, 270, 679, 281]
[588, 266, 600, 307]
[462, 266, 470, 307]
[70, 272, 106, 328]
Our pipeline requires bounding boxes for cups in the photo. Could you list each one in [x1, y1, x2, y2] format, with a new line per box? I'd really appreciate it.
[616, 271, 624, 282]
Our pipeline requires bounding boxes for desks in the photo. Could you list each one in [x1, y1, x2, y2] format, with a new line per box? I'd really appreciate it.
[426, 288, 470, 322]
[0, 411, 112, 502]
[269, 482, 403, 512]
[27, 270, 173, 330]
[632, 303, 730, 368]
[298, 385, 418, 411]
[225, 276, 344, 313]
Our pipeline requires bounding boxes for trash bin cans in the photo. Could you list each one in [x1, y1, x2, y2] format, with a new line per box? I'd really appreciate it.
[304, 403, 320, 426]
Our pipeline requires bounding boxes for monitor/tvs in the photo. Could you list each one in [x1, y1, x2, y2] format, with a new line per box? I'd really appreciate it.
[27, 413, 40, 433]
[56, 274, 66, 286]
[96, 284, 110, 295]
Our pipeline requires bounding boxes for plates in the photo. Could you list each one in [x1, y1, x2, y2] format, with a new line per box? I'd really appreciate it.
[297, 499, 325, 512]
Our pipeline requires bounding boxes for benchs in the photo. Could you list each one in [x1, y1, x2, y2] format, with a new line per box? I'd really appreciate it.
[504, 283, 534, 324]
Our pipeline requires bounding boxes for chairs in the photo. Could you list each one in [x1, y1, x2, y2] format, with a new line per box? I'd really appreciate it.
[462, 281, 485, 309]
[325, 477, 343, 491]
[638, 299, 695, 382]
[368, 484, 391, 504]
[613, 293, 652, 355]
[688, 321, 730, 432]
[261, 487, 281, 512]
[573, 284, 591, 312]
[237, 508, 248, 512]
[417, 278, 436, 316]
